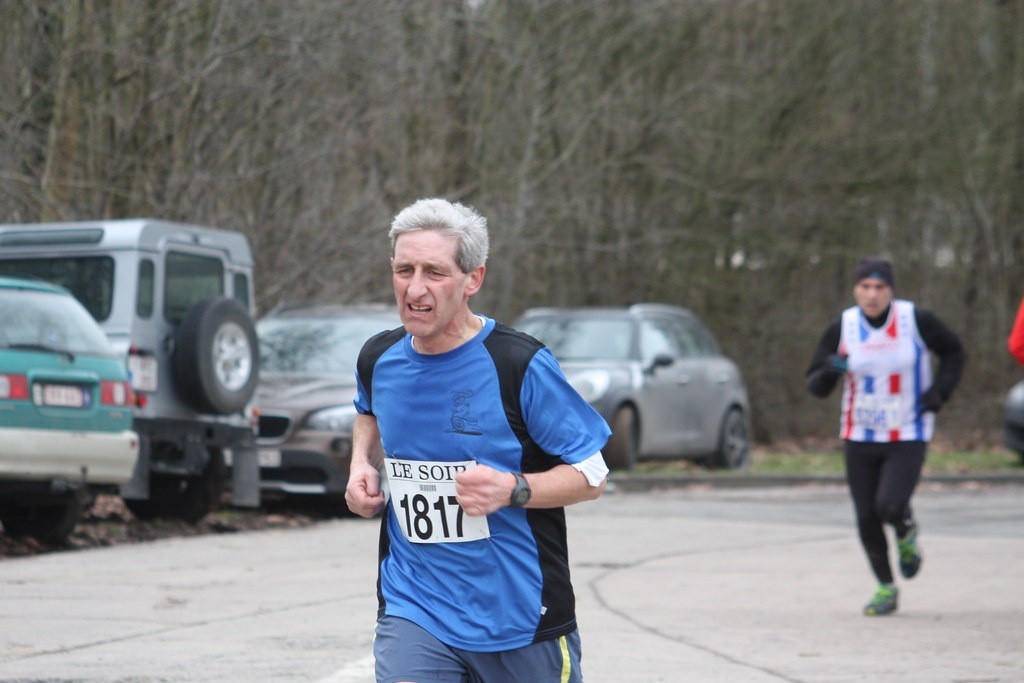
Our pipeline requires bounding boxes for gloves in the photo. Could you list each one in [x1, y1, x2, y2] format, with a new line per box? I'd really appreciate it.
[917, 393, 943, 415]
[825, 353, 849, 375]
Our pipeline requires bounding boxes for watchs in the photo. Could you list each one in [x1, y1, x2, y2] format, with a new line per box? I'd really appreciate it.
[509, 472, 531, 508]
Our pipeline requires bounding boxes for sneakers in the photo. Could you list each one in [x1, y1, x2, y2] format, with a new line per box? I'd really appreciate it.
[865, 584, 897, 617]
[896, 522, 922, 577]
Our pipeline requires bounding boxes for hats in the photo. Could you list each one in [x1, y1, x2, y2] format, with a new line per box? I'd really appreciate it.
[854, 255, 893, 284]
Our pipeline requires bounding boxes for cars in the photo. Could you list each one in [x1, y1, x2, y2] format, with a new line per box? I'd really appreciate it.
[0, 274, 141, 545]
[244, 301, 401, 520]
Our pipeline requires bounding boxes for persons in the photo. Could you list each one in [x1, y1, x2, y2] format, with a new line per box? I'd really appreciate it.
[804, 258, 965, 617]
[344, 200, 614, 683]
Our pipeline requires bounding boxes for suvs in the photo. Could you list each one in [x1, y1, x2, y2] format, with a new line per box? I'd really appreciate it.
[509, 299, 753, 472]
[0, 218, 261, 521]
